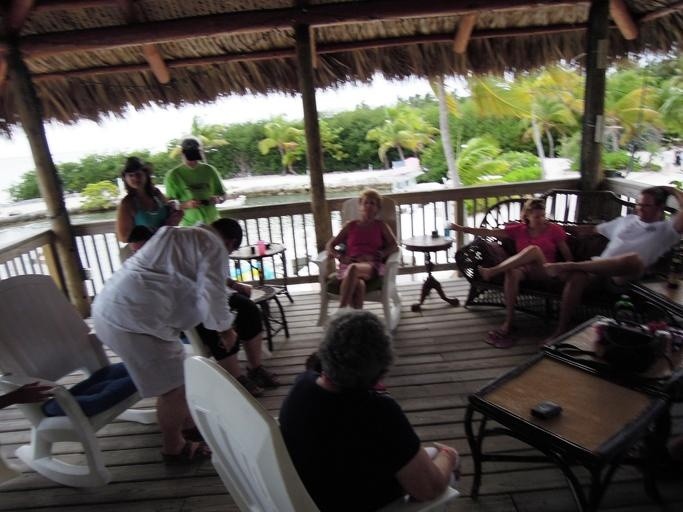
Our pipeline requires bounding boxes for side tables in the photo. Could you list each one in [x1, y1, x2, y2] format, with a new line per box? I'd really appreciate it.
[624, 278, 683, 329]
[248, 285, 290, 347]
[399, 235, 460, 311]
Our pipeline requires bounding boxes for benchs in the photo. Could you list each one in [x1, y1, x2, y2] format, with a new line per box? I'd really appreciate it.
[455, 188, 683, 328]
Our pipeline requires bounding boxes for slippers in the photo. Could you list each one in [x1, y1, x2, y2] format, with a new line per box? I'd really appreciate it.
[162, 438, 211, 461]
[496, 336, 519, 348]
[486, 328, 510, 344]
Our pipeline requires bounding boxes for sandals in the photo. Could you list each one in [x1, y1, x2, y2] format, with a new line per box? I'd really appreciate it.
[233, 375, 265, 398]
[245, 364, 280, 387]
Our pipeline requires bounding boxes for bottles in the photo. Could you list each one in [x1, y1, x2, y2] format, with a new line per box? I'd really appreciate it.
[667, 247, 682, 289]
[613, 295, 634, 326]
[444, 217, 450, 238]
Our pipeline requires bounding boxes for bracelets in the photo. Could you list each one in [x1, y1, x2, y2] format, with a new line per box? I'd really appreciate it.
[439, 446, 457, 464]
[218, 324, 237, 336]
[230, 280, 238, 288]
[377, 249, 384, 258]
[218, 196, 224, 204]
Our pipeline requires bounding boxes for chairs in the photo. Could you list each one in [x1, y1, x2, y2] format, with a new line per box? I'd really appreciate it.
[0, 274, 160, 490]
[307, 197, 402, 333]
[182, 355, 462, 511]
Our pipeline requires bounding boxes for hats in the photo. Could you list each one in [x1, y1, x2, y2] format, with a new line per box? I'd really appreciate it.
[182, 139, 203, 161]
[121, 157, 154, 181]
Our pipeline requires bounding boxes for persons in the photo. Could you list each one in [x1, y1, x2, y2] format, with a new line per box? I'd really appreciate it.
[164, 138, 229, 228]
[127, 222, 280, 398]
[447, 197, 573, 339]
[536, 185, 683, 351]
[112, 156, 185, 255]
[325, 188, 400, 321]
[278, 307, 462, 511]
[89, 217, 243, 465]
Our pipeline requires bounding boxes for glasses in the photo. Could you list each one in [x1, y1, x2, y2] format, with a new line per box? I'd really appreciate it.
[634, 203, 655, 209]
[529, 200, 545, 205]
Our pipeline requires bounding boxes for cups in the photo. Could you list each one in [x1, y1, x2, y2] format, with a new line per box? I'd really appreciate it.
[258, 240, 266, 256]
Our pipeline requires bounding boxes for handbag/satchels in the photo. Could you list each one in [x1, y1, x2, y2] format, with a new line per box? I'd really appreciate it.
[594, 318, 668, 371]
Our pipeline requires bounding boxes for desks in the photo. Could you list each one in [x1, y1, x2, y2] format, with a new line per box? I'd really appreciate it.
[463, 351, 666, 512]
[229, 242, 294, 304]
[538, 314, 683, 481]
